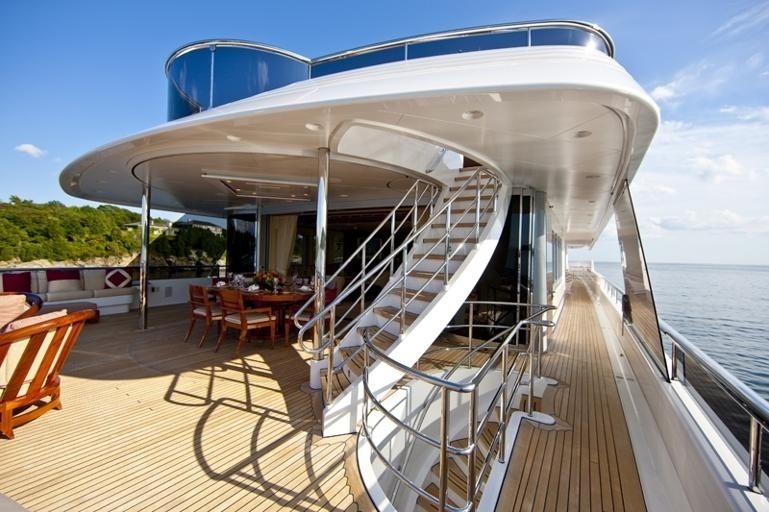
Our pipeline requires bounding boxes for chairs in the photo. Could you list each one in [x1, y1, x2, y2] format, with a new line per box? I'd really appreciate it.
[185, 274, 345, 359]
[0, 291, 96, 440]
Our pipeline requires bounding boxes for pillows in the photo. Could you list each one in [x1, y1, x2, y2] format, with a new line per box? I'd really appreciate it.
[2, 265, 133, 294]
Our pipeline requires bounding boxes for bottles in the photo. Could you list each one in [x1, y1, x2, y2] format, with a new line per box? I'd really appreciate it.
[272, 273, 280, 295]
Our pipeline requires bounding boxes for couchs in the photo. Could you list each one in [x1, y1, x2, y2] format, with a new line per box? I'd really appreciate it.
[0, 268, 138, 316]
